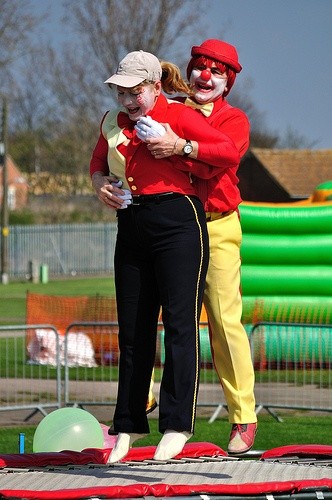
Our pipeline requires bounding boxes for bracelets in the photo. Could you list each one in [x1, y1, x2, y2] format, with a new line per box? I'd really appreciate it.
[175, 138, 180, 155]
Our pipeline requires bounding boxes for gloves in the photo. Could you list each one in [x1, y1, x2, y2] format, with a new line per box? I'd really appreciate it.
[134, 116, 165, 143]
[109, 181, 133, 210]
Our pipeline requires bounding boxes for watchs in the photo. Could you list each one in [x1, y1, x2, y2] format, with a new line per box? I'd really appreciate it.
[183, 139, 193, 157]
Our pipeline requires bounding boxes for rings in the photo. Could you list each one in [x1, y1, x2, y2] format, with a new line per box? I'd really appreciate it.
[105, 197, 107, 200]
[154, 151, 156, 155]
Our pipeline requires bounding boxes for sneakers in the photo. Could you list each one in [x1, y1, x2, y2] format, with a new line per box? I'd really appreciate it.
[228, 421, 259, 454]
[108, 398, 157, 436]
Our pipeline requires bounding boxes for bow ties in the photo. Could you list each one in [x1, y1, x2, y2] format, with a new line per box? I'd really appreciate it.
[185, 98, 214, 118]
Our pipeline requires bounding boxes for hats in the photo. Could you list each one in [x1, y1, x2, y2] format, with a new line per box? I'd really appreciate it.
[102, 50, 162, 87]
[192, 39, 242, 73]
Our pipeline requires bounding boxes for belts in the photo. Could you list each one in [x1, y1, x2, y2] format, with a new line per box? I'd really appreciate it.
[133, 194, 185, 205]
[206, 210, 233, 222]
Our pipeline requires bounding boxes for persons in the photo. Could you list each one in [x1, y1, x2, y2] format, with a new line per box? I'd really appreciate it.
[89, 50, 240, 464]
[111, 39, 258, 454]
